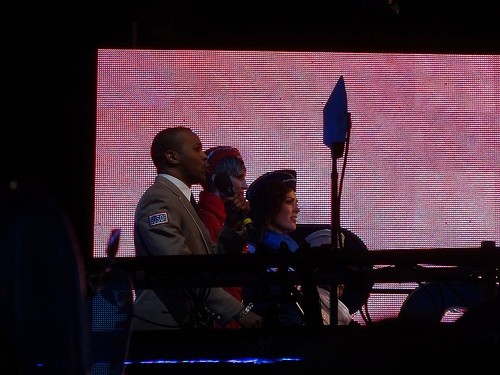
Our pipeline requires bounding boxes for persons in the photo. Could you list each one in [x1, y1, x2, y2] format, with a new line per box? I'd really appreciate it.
[239, 169, 317, 328]
[121, 127, 263, 375]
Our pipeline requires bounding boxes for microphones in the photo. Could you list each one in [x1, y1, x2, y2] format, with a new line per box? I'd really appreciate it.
[213, 172, 253, 230]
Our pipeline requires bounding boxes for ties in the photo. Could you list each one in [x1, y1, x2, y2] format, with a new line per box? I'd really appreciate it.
[190, 193, 200, 216]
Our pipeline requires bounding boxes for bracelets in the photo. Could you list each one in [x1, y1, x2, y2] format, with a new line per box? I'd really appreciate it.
[237, 302, 253, 321]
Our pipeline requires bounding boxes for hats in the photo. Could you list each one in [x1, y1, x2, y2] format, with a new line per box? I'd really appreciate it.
[201, 146, 242, 190]
[246, 169, 296, 207]
